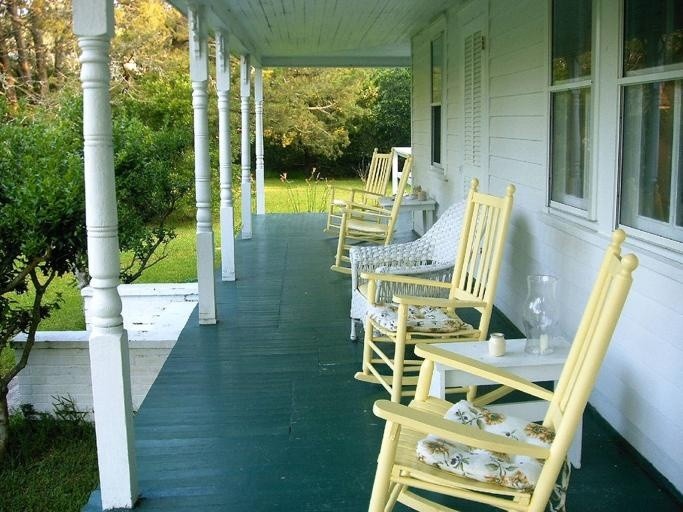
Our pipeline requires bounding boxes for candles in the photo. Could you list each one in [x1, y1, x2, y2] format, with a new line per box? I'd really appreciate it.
[541, 335, 549, 355]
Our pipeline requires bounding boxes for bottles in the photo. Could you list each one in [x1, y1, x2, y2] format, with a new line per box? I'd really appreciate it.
[488, 333, 505, 357]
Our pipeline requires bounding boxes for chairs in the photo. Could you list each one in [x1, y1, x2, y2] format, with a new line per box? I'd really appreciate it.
[349, 201, 489, 339]
[330, 157, 413, 275]
[324, 148, 394, 238]
[368, 229, 639, 512]
[354, 178, 516, 402]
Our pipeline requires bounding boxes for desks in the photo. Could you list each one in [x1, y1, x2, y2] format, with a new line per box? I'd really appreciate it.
[392, 147, 412, 194]
[378, 197, 436, 236]
[422, 339, 583, 469]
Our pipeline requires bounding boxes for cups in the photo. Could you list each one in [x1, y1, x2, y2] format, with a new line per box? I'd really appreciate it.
[418, 192, 426, 200]
